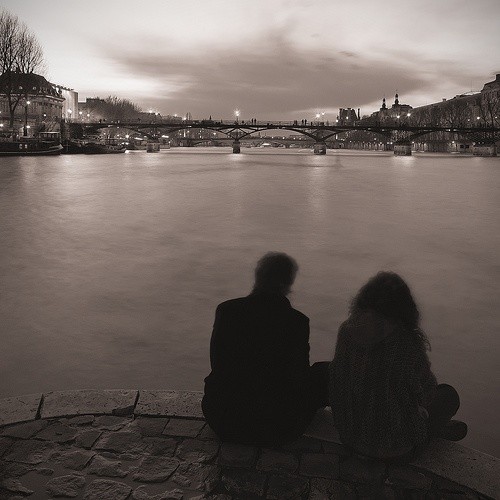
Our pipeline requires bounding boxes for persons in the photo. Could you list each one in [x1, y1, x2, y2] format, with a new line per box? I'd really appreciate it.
[200, 251, 334, 437]
[331, 269, 467, 460]
